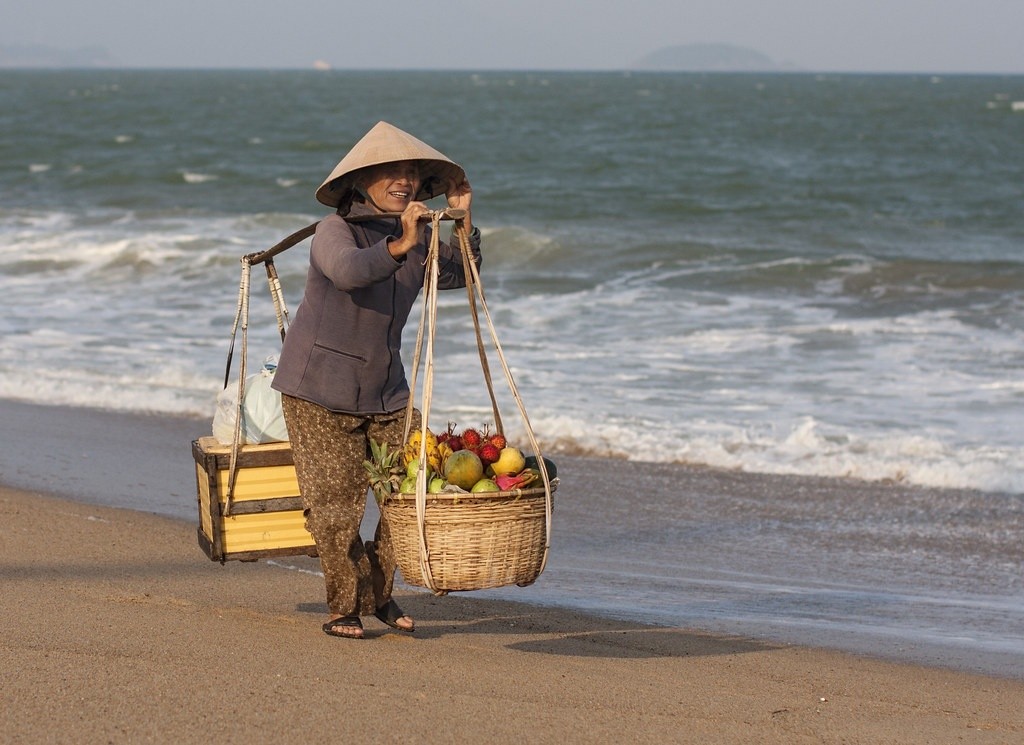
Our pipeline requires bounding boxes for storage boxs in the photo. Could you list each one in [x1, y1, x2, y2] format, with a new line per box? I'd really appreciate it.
[192, 435, 323, 565]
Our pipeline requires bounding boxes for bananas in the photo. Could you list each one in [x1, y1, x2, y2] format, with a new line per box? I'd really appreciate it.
[401, 425, 454, 477]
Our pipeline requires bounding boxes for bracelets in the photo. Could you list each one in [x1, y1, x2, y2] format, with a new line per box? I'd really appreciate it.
[451, 223, 475, 238]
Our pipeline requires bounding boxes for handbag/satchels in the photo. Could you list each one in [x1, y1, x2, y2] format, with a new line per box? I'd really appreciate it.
[211, 355, 294, 445]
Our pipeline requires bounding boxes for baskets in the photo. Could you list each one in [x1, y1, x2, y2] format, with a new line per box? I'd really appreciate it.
[380, 481, 558, 591]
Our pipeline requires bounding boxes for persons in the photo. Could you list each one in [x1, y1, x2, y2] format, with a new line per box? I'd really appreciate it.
[271, 122, 482, 638]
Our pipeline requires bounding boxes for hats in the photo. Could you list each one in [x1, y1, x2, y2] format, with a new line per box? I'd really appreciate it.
[314, 120, 466, 208]
